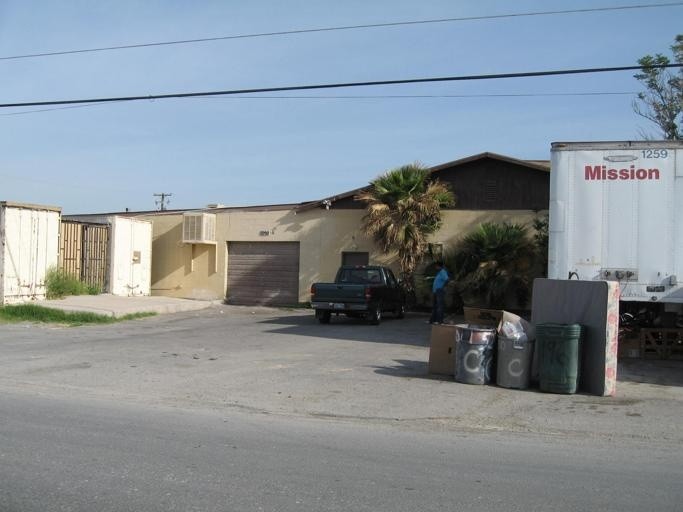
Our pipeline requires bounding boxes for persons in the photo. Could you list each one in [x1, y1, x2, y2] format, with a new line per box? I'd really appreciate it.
[425, 262, 448, 325]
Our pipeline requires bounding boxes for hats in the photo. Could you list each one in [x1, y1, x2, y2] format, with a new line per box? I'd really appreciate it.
[434, 260, 444, 267]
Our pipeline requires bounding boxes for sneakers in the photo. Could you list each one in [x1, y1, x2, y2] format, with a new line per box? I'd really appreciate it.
[425, 320, 431, 323]
[433, 322, 439, 324]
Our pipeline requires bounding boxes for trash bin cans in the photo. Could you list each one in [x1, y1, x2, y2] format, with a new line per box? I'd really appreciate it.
[452, 325, 497, 386]
[494, 334, 534, 391]
[535, 321, 583, 396]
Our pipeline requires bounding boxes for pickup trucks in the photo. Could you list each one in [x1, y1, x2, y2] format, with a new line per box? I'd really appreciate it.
[311, 265, 406, 324]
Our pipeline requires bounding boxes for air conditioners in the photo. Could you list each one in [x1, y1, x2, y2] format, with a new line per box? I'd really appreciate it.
[183, 212, 217, 246]
